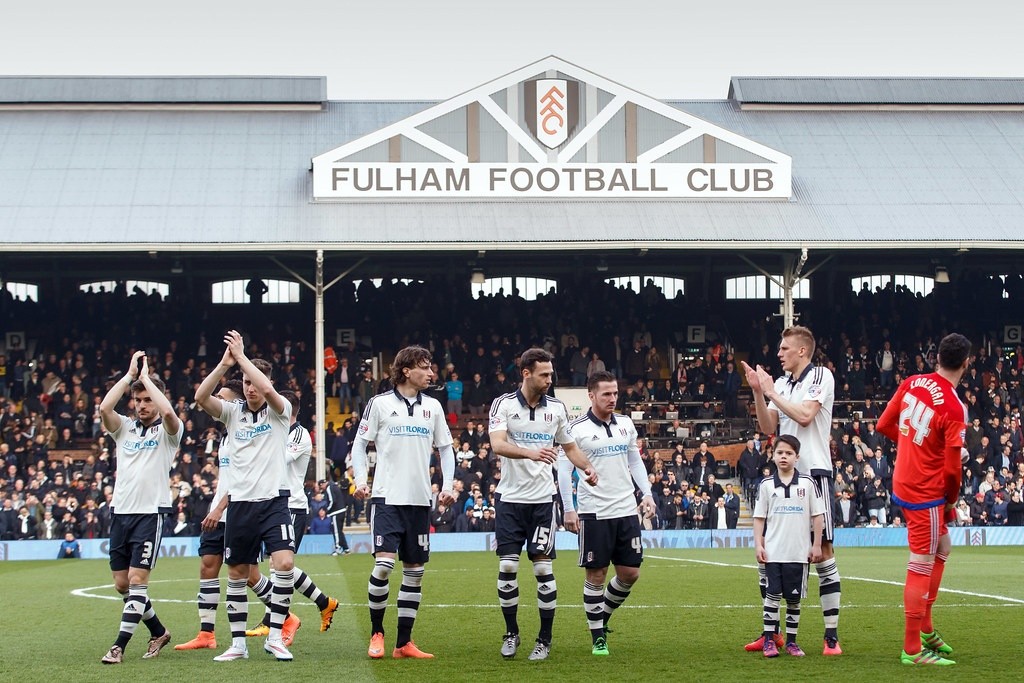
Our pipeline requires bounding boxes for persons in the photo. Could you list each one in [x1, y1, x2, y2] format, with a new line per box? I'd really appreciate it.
[350, 346, 455, 660]
[86, 350, 173, 664]
[557, 370, 658, 655]
[173, 328, 338, 662]
[488, 347, 600, 661]
[741, 325, 844, 658]
[876, 334, 971, 668]
[0, 261, 1024, 560]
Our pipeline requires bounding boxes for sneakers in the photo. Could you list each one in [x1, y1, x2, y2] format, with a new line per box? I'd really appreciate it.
[823, 635, 843, 655]
[264, 637, 294, 661]
[102, 645, 123, 664]
[317, 596, 338, 632]
[281, 611, 301, 646]
[762, 635, 780, 657]
[212, 644, 249, 661]
[744, 630, 784, 651]
[500, 631, 520, 658]
[245, 623, 270, 636]
[586, 620, 615, 640]
[900, 646, 956, 667]
[529, 638, 552, 660]
[174, 631, 216, 650]
[784, 642, 806, 657]
[590, 637, 610, 655]
[393, 640, 434, 660]
[367, 632, 385, 659]
[920, 630, 953, 656]
[143, 628, 171, 659]
